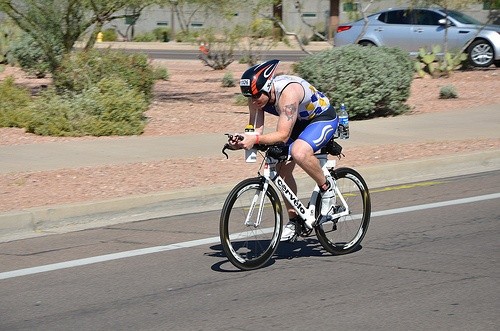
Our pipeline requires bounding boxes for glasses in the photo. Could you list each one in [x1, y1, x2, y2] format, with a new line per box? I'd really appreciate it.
[249, 93, 262, 99]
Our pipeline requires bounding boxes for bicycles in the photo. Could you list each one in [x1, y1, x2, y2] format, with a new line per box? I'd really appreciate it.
[219, 133, 372, 270]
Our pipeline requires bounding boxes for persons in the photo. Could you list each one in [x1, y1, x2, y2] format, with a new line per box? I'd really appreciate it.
[227, 59, 338, 242]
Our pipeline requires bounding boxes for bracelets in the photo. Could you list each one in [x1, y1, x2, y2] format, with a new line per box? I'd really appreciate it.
[256, 135, 259, 145]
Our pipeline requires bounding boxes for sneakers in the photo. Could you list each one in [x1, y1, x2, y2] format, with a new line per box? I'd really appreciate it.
[280, 221, 296, 241]
[320, 175, 335, 217]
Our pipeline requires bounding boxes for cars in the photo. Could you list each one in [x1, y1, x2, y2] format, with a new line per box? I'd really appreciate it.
[333, 7, 500, 67]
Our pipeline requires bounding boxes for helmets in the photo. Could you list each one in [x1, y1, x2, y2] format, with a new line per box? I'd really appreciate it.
[239, 59, 280, 96]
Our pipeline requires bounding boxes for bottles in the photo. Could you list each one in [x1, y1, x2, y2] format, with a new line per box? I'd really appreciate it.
[244, 125, 256, 163]
[338, 106, 349, 139]
